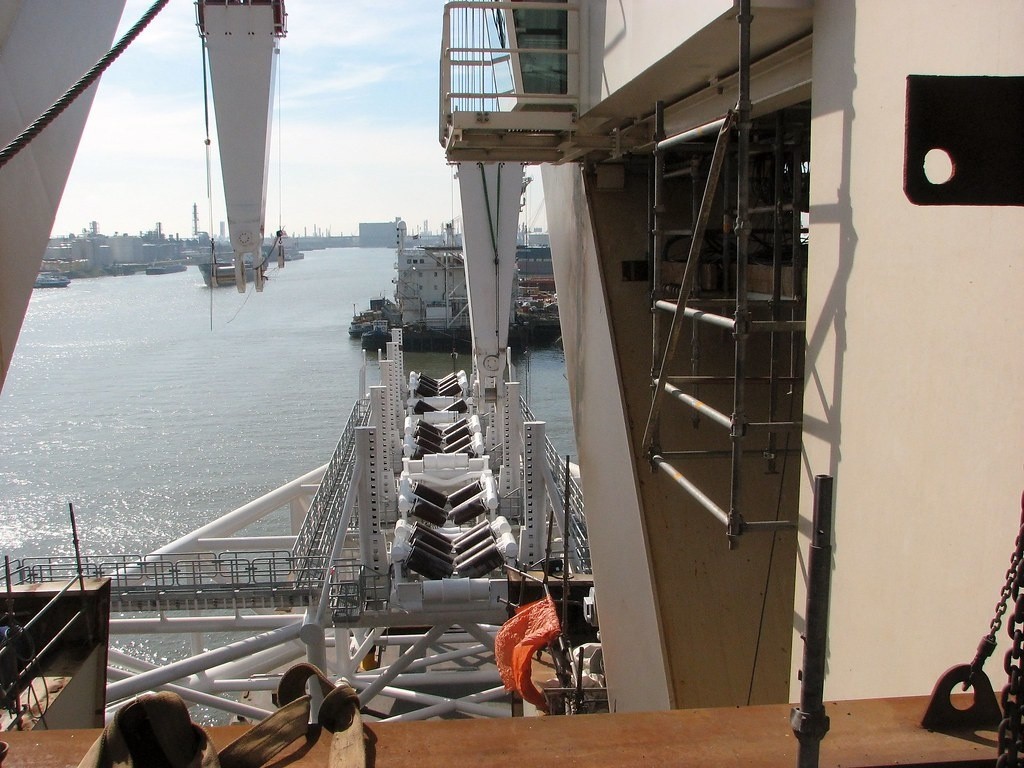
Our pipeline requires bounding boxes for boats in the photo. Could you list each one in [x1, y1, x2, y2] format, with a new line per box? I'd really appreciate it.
[348, 295, 393, 353]
[198, 257, 269, 287]
[33, 273, 71, 288]
[144, 263, 188, 274]
